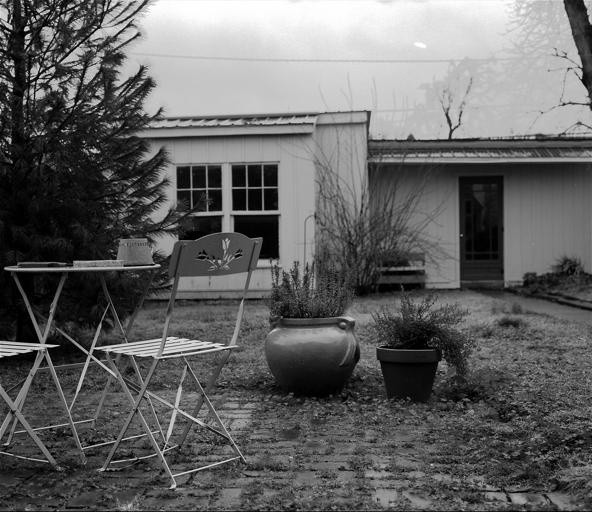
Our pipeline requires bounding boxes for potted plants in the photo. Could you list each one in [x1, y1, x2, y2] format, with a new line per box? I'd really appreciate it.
[265, 257, 471, 403]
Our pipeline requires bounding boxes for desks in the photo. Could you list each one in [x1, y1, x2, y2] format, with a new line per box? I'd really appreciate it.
[5, 259, 164, 468]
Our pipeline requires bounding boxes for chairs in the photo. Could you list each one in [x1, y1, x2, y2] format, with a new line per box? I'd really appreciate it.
[0, 340, 66, 475]
[92, 233, 264, 489]
